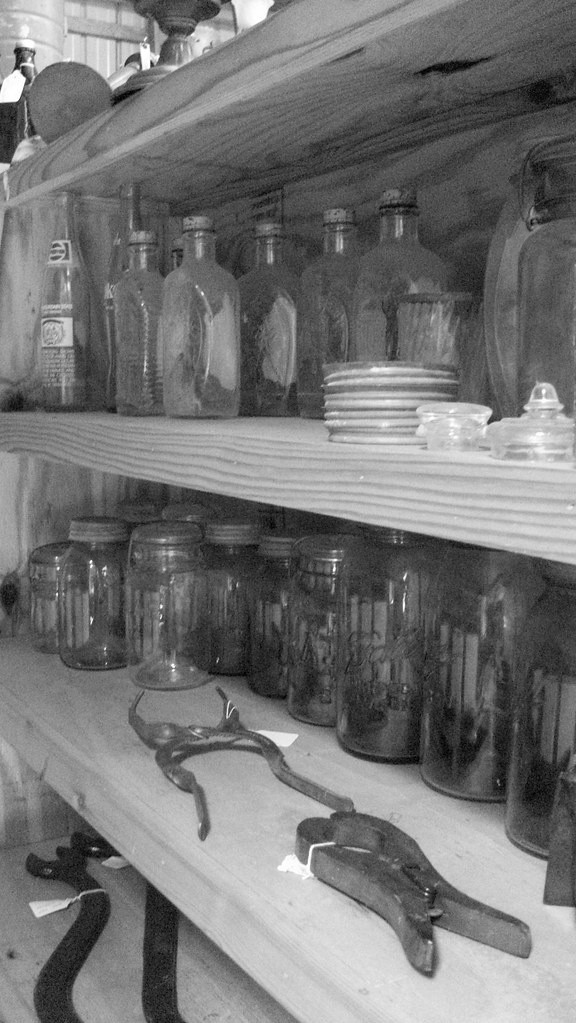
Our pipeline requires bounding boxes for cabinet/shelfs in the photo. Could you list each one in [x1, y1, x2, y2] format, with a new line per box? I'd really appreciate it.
[0, 0, 576, 1023]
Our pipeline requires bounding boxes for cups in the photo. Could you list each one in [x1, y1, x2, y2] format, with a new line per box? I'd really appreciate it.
[396, 292, 487, 406]
[416, 402, 492, 450]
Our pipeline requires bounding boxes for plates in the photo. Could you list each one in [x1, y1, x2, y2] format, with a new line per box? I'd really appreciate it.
[319, 361, 460, 445]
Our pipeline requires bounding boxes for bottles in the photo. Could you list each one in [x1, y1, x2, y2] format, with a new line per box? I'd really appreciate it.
[512, 134, 575, 422]
[35, 189, 90, 413]
[101, 181, 448, 419]
[28, 498, 574, 862]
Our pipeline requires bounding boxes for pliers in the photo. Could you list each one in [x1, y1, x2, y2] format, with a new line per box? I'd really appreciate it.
[128, 685, 356, 842]
[295, 810, 532, 979]
